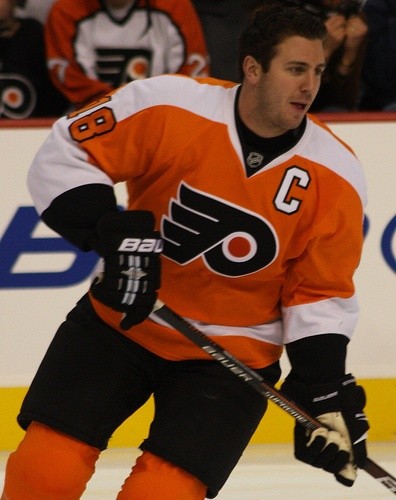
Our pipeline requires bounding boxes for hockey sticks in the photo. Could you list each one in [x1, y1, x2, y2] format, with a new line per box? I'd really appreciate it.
[149, 299, 396, 496]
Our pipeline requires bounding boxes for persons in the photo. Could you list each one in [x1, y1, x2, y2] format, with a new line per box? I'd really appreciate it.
[0, 5, 369, 500]
[44, 0, 369, 120]
[0, 0, 69, 121]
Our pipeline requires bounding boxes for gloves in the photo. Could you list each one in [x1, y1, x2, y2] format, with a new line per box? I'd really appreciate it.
[90, 209, 163, 333]
[292, 374, 371, 487]
